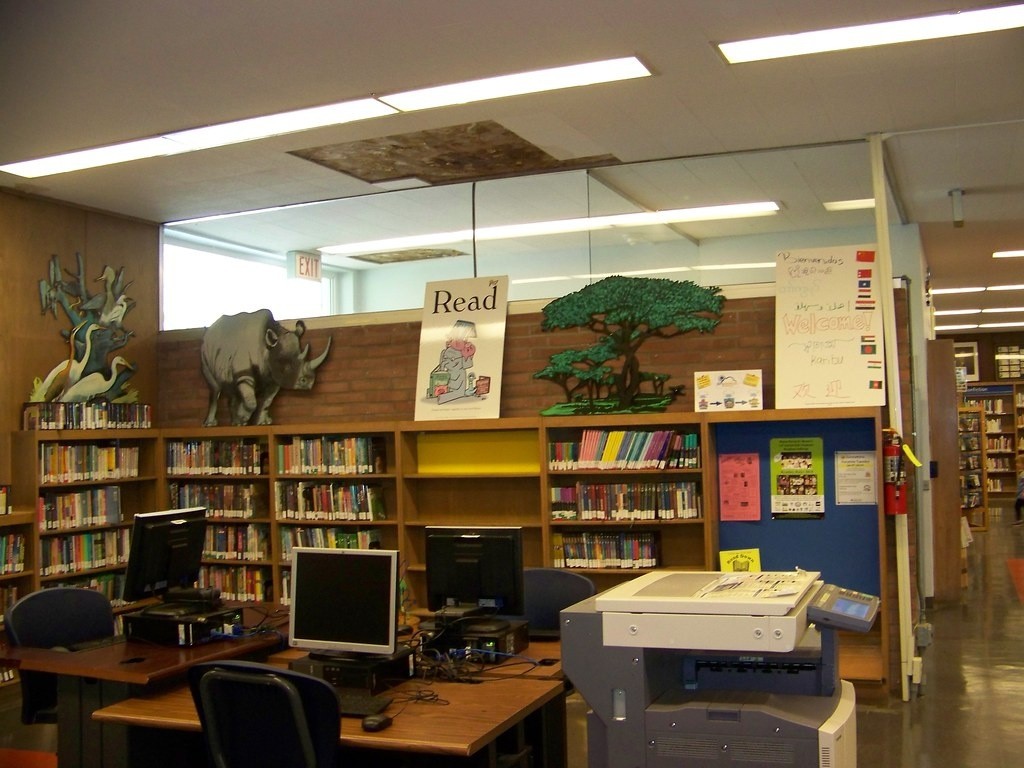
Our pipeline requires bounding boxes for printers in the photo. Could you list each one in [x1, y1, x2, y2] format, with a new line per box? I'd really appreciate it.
[560, 570, 880, 768]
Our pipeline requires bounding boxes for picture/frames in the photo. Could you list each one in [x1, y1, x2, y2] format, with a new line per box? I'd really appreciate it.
[955, 341, 979, 383]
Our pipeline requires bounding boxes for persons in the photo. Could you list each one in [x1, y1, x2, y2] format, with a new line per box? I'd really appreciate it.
[777, 475, 817, 495]
[1012, 477, 1024, 526]
[782, 452, 812, 468]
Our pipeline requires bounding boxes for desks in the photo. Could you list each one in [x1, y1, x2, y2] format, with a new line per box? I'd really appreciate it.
[0, 603, 290, 768]
[91, 628, 566, 768]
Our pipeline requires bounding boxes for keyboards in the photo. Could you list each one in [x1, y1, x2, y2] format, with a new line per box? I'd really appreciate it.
[63, 634, 126, 654]
[337, 692, 394, 718]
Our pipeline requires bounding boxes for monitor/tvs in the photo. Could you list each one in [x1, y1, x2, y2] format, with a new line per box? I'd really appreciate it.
[288, 546, 400, 662]
[123, 507, 209, 616]
[425, 526, 524, 632]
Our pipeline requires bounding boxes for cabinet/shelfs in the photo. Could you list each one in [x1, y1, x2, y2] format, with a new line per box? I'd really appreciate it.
[0, 277, 1024, 704]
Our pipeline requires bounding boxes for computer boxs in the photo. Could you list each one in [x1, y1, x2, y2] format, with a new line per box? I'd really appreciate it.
[123, 605, 244, 648]
[288, 645, 417, 695]
[418, 621, 529, 665]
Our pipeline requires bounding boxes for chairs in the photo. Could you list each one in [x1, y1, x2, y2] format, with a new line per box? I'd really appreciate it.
[4, 585, 117, 727]
[185, 661, 344, 768]
[494, 567, 595, 637]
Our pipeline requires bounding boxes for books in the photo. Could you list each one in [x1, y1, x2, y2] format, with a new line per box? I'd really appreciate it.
[39, 486, 139, 636]
[548, 428, 701, 570]
[166, 441, 269, 602]
[38, 442, 139, 484]
[0, 484, 25, 624]
[1016, 392, 1024, 450]
[22, 401, 152, 430]
[275, 436, 387, 606]
[957, 399, 1012, 510]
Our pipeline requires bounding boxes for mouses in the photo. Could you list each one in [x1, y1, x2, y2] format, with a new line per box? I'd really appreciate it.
[52, 644, 79, 654]
[362, 714, 393, 731]
[397, 624, 413, 636]
